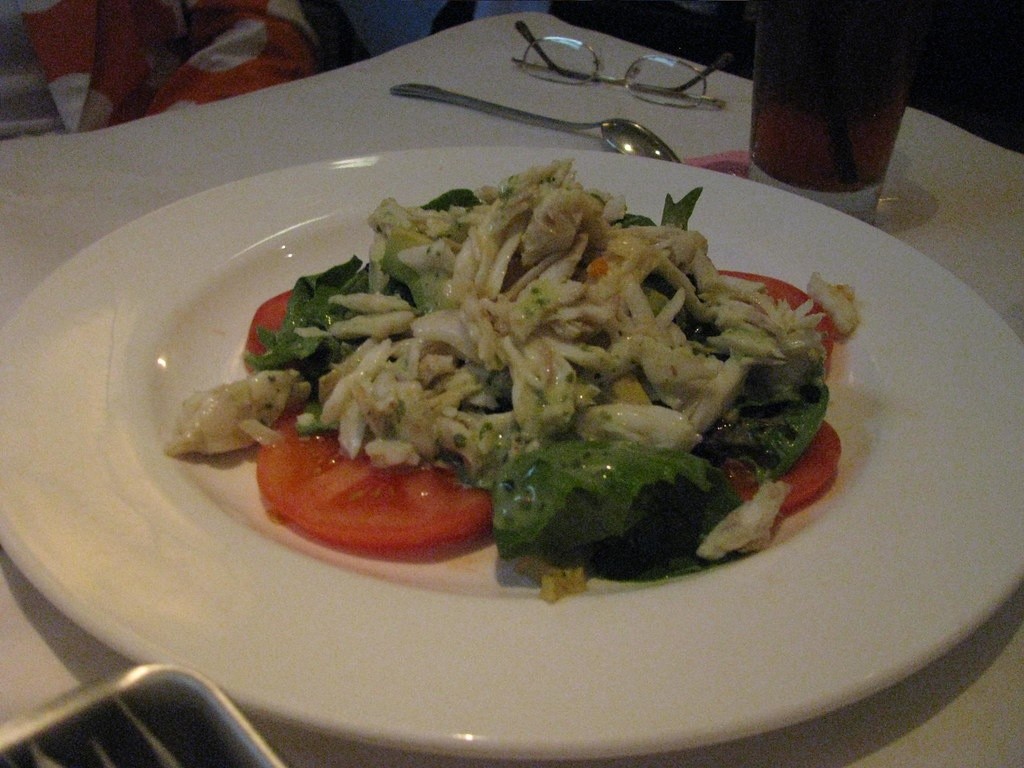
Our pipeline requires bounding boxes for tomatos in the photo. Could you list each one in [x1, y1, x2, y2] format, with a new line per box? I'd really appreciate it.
[255, 411, 492, 560]
[739, 419, 841, 510]
[718, 269, 833, 369]
[245, 291, 292, 359]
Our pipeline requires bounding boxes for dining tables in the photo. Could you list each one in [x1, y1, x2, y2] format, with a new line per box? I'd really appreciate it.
[0, 11, 1024, 768]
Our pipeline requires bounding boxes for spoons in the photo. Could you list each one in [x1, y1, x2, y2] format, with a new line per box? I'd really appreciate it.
[390, 83, 682, 164]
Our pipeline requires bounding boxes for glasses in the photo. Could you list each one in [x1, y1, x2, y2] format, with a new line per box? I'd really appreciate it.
[512, 20, 722, 110]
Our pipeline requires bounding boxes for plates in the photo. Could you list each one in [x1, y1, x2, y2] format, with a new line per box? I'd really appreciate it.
[0, 143, 1024, 760]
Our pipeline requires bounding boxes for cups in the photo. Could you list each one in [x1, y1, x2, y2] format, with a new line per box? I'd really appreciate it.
[748, 0, 922, 225]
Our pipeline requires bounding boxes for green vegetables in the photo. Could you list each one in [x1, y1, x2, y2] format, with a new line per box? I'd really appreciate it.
[256, 187, 828, 582]
